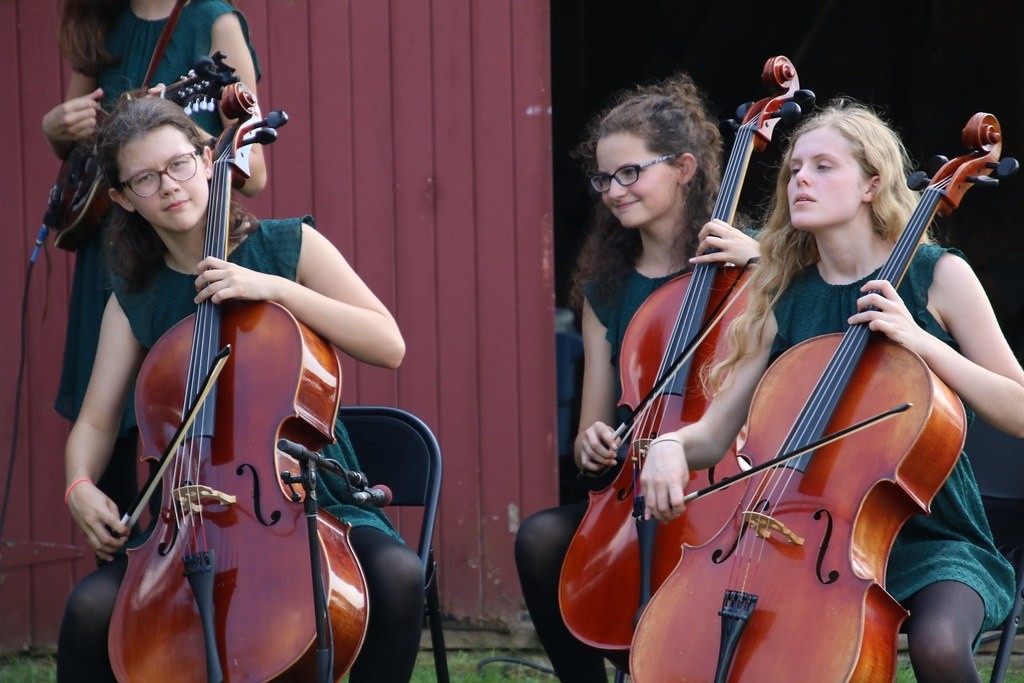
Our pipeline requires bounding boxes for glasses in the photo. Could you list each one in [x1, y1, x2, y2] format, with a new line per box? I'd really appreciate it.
[116, 149, 205, 198]
[589, 153, 682, 193]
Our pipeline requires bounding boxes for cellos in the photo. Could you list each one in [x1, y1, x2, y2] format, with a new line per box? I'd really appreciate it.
[557, 52, 816, 676]
[109, 80, 366, 682]
[627, 110, 1018, 683]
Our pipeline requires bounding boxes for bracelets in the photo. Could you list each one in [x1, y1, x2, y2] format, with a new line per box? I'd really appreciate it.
[64, 478, 92, 504]
[646, 439, 684, 451]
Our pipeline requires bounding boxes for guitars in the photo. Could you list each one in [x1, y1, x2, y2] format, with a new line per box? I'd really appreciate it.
[49, 50, 241, 253]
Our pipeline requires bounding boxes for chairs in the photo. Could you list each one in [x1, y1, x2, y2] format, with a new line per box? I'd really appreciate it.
[961, 414, 1024, 683]
[337, 407, 451, 683]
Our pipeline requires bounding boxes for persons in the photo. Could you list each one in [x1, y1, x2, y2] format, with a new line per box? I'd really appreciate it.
[44, 0, 426, 682]
[510, 73, 1024, 683]
